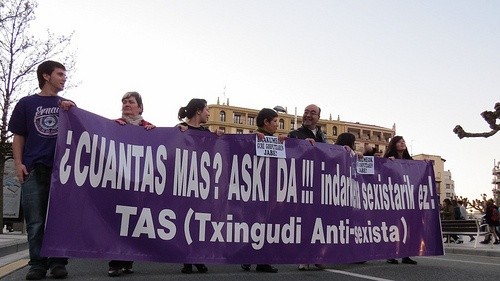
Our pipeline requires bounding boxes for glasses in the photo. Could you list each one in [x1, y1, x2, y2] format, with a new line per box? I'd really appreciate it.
[51, 72, 67, 80]
[304, 111, 320, 116]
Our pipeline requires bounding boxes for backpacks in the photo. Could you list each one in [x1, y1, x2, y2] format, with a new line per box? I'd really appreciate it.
[490, 208, 500, 221]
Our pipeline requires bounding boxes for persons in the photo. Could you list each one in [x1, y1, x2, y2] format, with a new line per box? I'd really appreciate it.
[173, 98, 224, 274]
[334, 133, 377, 264]
[480, 198, 500, 244]
[287, 104, 328, 271]
[8, 60, 77, 281]
[108, 92, 156, 277]
[383, 136, 417, 265]
[440, 198, 475, 244]
[241, 108, 288, 273]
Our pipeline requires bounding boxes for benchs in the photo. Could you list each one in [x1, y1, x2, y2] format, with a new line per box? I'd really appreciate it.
[441, 219, 495, 248]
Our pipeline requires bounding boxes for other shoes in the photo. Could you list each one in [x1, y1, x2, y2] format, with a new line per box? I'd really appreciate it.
[182, 265, 192, 273]
[196, 266, 208, 273]
[445, 238, 464, 244]
[26, 264, 47, 280]
[256, 264, 279, 273]
[298, 264, 309, 270]
[468, 237, 476, 242]
[49, 264, 68, 279]
[108, 268, 134, 277]
[385, 259, 399, 264]
[241, 265, 251, 270]
[481, 238, 500, 244]
[400, 258, 417, 265]
